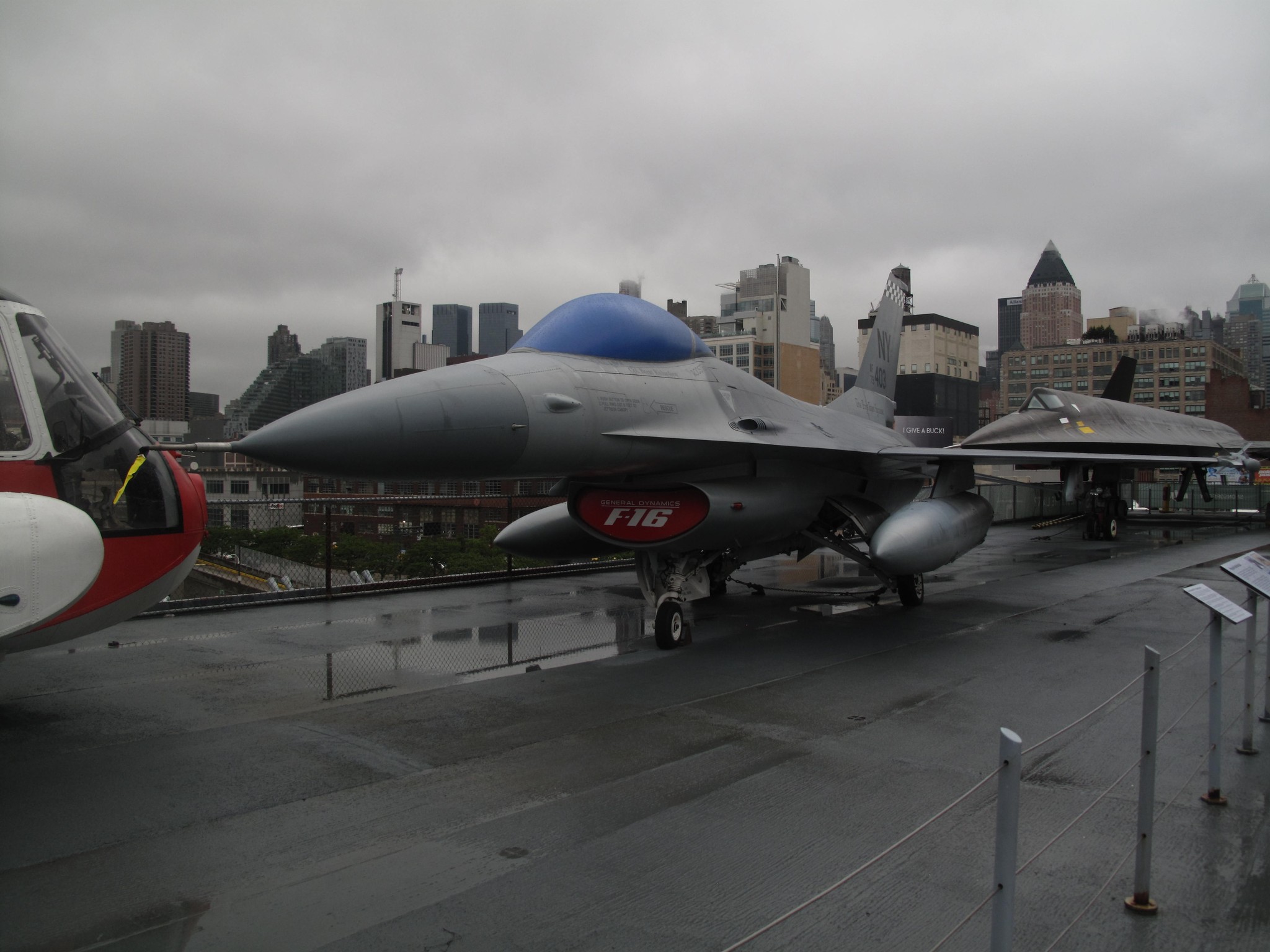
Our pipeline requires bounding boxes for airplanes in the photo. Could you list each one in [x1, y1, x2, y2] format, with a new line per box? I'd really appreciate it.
[941, 356, 1261, 542]
[139, 264, 995, 651]
[0, 286, 208, 655]
